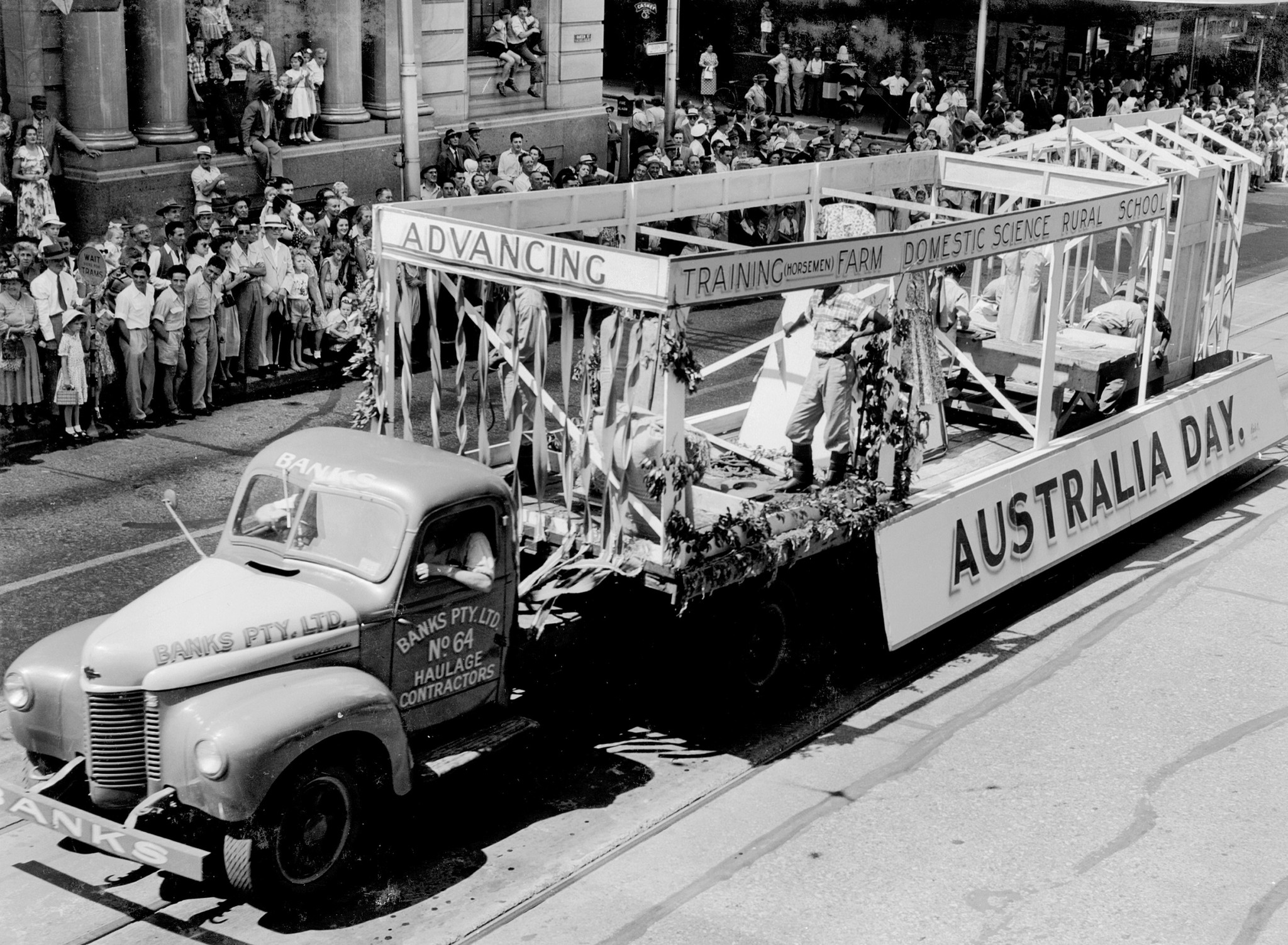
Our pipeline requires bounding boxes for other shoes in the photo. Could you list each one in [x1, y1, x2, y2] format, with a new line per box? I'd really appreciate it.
[0, 411, 217, 440]
[527, 86, 541, 97]
[497, 78, 520, 97]
[531, 48, 546, 55]
[221, 353, 323, 386]
[703, 100, 713, 106]
[289, 131, 322, 146]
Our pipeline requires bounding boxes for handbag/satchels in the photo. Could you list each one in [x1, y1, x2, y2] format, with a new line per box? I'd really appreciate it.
[222, 285, 236, 308]
[704, 66, 713, 79]
[0, 329, 27, 372]
[271, 300, 293, 344]
[55, 384, 79, 406]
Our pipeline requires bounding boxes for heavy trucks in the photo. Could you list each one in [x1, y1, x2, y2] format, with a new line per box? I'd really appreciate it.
[0, 100, 1288, 914]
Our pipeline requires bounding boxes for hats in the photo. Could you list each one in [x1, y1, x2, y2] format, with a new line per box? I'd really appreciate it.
[110, 197, 288, 231]
[0, 214, 91, 330]
[254, 83, 281, 99]
[420, 122, 595, 192]
[192, 145, 212, 157]
[0, 92, 47, 107]
[1137, 294, 1165, 313]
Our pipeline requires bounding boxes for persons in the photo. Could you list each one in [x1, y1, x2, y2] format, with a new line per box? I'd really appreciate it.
[929, 261, 970, 378]
[253, 491, 318, 547]
[415, 516, 496, 592]
[968, 274, 1005, 389]
[774, 285, 893, 492]
[488, 285, 550, 496]
[1077, 295, 1171, 418]
[0, 0, 1288, 445]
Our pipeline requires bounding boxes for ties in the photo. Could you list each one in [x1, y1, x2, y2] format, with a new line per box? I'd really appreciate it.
[37, 122, 42, 144]
[57, 274, 67, 311]
[607, 122, 613, 133]
[255, 41, 263, 72]
[789, 220, 795, 240]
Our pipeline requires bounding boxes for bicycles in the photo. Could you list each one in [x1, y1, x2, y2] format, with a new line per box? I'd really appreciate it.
[712, 80, 774, 117]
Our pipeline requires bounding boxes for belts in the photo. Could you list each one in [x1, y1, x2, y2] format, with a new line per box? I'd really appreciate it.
[815, 351, 846, 358]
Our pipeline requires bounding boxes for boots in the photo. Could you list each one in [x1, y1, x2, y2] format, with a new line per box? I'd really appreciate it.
[773, 442, 850, 491]
[504, 444, 536, 496]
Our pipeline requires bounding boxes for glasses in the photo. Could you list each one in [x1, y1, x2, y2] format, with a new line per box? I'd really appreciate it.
[36, 105, 47, 110]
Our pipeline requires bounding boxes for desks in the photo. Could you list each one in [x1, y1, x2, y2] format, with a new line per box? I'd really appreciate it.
[943, 329, 1137, 432]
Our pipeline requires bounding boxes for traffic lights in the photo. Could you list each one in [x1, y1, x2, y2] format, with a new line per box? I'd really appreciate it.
[1031, 26, 1051, 66]
[839, 68, 866, 115]
[1014, 25, 1032, 68]
